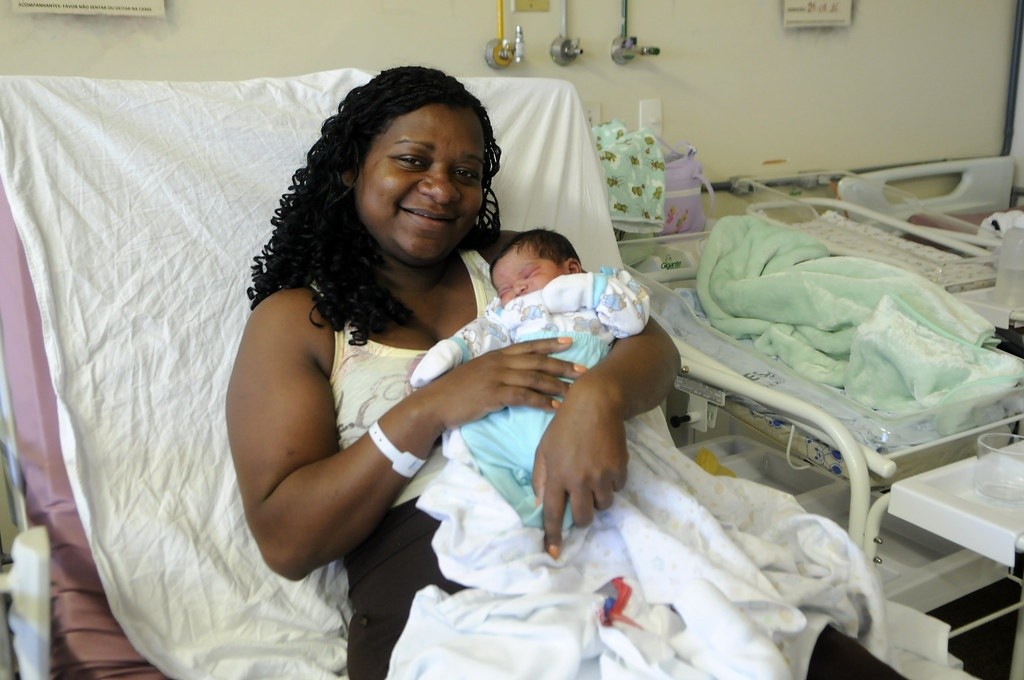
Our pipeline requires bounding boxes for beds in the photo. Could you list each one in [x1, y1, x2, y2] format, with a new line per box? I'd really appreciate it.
[0, 73, 1024, 680]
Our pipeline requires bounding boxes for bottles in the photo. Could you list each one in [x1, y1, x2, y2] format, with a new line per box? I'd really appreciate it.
[996, 215, 1024, 308]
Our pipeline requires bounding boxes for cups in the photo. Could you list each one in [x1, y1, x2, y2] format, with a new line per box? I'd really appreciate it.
[975, 433, 1024, 508]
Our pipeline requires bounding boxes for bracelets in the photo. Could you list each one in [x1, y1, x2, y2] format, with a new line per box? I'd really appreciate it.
[369, 420, 427, 478]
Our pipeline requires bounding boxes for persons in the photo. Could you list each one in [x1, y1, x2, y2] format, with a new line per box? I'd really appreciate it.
[226, 66, 908, 679]
[409, 228, 648, 530]
